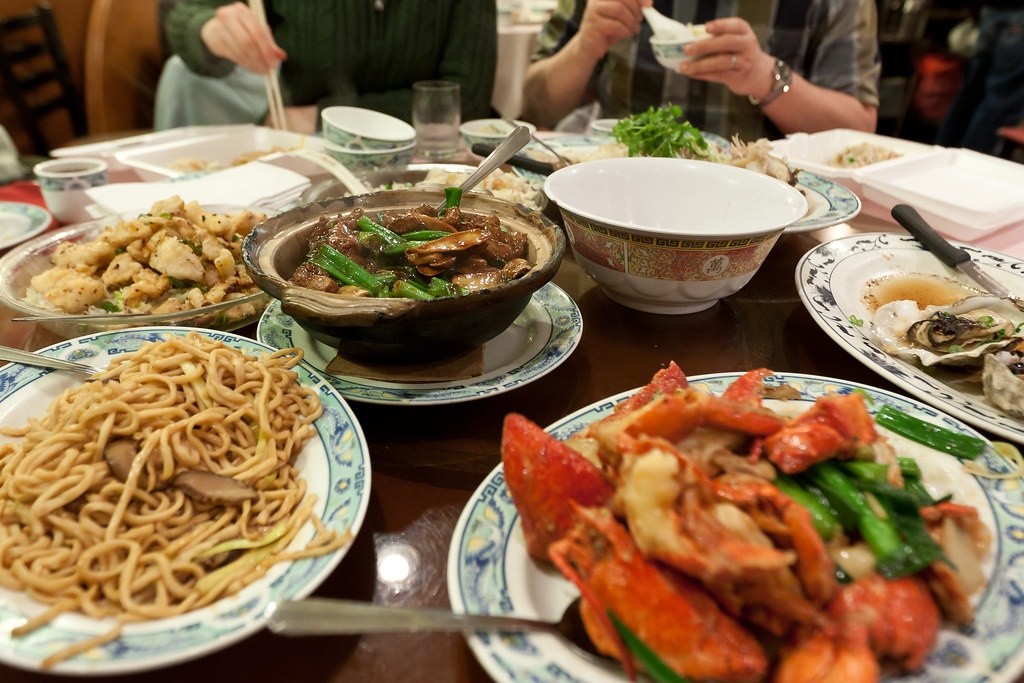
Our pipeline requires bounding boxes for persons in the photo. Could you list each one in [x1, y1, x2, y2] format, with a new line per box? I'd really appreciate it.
[935, 0, 1024, 155]
[520, 1, 882, 143]
[162, 0, 498, 143]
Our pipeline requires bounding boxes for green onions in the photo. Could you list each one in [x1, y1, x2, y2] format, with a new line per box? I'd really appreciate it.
[308, 185, 470, 304]
[849, 316, 1024, 354]
[608, 400, 988, 683]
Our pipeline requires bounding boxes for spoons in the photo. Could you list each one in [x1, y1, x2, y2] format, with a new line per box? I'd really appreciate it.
[260, 594, 625, 664]
[642, 6, 692, 41]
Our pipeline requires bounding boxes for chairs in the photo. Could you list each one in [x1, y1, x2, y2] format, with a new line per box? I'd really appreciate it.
[0, 0, 161, 156]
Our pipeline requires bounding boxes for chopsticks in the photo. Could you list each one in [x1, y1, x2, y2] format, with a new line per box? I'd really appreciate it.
[247, 0, 286, 130]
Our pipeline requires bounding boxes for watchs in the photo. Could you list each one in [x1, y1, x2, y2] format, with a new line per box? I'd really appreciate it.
[747, 54, 793, 109]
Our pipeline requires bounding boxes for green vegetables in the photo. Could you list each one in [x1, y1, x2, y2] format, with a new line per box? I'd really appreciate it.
[611, 103, 708, 159]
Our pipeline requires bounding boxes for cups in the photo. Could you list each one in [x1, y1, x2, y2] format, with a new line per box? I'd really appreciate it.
[33, 158, 109, 225]
[411, 79, 462, 159]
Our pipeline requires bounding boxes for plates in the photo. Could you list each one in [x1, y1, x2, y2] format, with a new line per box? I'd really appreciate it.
[0, 326, 372, 678]
[256, 282, 583, 405]
[510, 137, 862, 236]
[695, 131, 731, 158]
[795, 232, 1024, 444]
[445, 371, 1024, 682]
[0, 198, 53, 251]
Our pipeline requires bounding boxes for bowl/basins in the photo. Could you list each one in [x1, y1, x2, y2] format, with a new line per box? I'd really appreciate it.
[0, 205, 286, 333]
[48, 123, 325, 186]
[243, 189, 566, 367]
[320, 105, 418, 173]
[590, 118, 622, 137]
[766, 126, 1024, 242]
[543, 157, 808, 316]
[649, 24, 715, 73]
[457, 118, 537, 161]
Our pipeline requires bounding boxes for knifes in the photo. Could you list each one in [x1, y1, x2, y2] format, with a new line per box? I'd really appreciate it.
[891, 205, 1015, 300]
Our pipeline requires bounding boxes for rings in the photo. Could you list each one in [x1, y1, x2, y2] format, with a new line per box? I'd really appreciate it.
[730, 53, 738, 70]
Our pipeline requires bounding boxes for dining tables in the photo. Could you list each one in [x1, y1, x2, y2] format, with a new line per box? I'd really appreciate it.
[492, 0, 558, 119]
[0, 121, 1024, 683]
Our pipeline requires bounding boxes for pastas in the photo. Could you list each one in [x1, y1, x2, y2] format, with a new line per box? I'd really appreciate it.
[0, 328, 352, 673]
